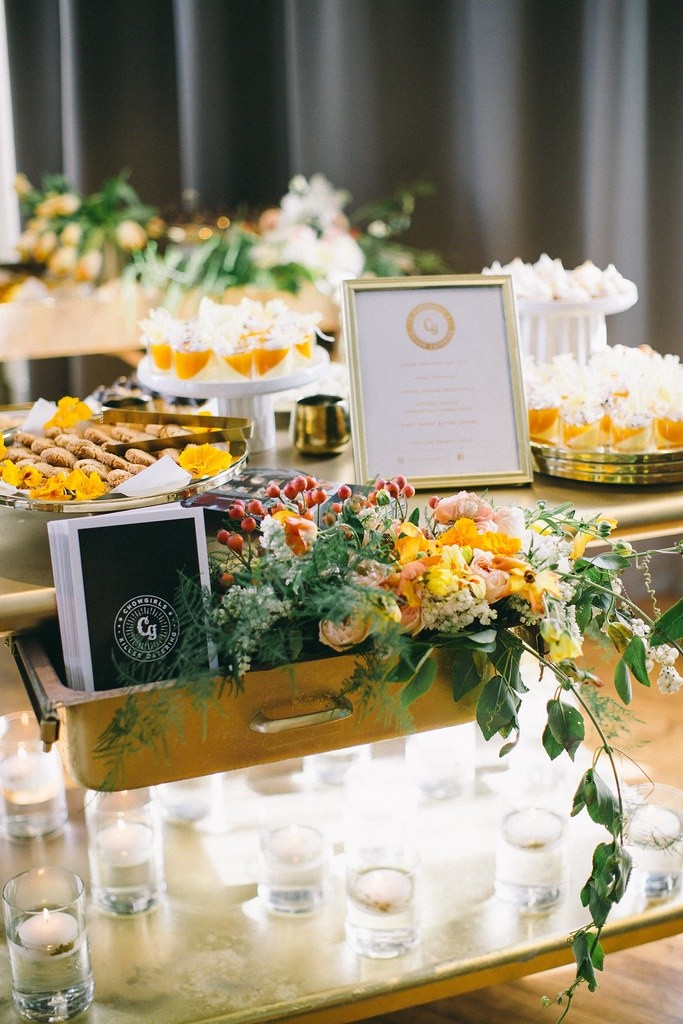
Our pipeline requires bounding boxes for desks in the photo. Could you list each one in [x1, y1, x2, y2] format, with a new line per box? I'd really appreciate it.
[0, 272, 377, 404]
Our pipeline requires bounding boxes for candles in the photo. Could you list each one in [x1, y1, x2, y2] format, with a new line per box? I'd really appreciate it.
[0, 709, 683, 1024]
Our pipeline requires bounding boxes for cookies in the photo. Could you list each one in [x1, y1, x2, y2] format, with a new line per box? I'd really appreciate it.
[6, 422, 194, 488]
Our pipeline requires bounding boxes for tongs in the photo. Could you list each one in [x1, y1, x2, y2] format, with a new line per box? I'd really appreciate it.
[103, 409, 252, 455]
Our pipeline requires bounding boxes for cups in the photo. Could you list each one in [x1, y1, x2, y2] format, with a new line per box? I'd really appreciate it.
[258, 821, 330, 913]
[3, 864, 95, 1024]
[622, 806, 682, 901]
[474, 719, 509, 774]
[157, 775, 210, 823]
[497, 806, 567, 917]
[143, 310, 316, 380]
[304, 747, 355, 786]
[347, 866, 416, 958]
[293, 396, 350, 455]
[524, 343, 683, 452]
[369, 737, 405, 774]
[0, 709, 69, 843]
[85, 786, 163, 914]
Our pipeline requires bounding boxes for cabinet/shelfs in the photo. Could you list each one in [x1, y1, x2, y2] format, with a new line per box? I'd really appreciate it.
[0, 428, 683, 1024]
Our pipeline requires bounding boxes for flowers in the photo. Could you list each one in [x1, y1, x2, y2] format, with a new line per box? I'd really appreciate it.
[11, 164, 164, 295]
[164, 173, 453, 299]
[79, 472, 683, 1024]
